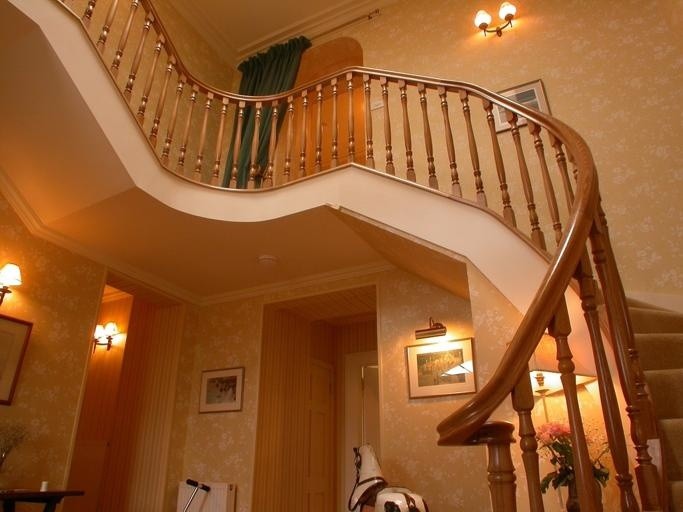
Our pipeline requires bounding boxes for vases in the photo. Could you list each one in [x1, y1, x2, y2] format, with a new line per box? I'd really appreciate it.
[566, 481, 602, 511]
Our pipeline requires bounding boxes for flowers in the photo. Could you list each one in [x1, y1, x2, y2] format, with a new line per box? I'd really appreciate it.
[535, 421, 609, 493]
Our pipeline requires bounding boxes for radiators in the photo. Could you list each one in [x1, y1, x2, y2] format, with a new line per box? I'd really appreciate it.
[177, 480, 236, 512]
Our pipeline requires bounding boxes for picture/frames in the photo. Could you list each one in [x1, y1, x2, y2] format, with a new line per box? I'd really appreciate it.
[407, 338, 478, 399]
[199, 367, 245, 414]
[491, 78, 551, 133]
[0, 314, 34, 405]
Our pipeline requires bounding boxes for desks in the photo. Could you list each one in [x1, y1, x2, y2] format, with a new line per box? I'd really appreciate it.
[1, 475, 85, 512]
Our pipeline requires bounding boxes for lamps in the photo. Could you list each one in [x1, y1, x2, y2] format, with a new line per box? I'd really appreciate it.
[416, 315, 448, 340]
[507, 334, 598, 511]
[92, 323, 119, 354]
[474, 2, 517, 37]
[0, 262, 22, 305]
